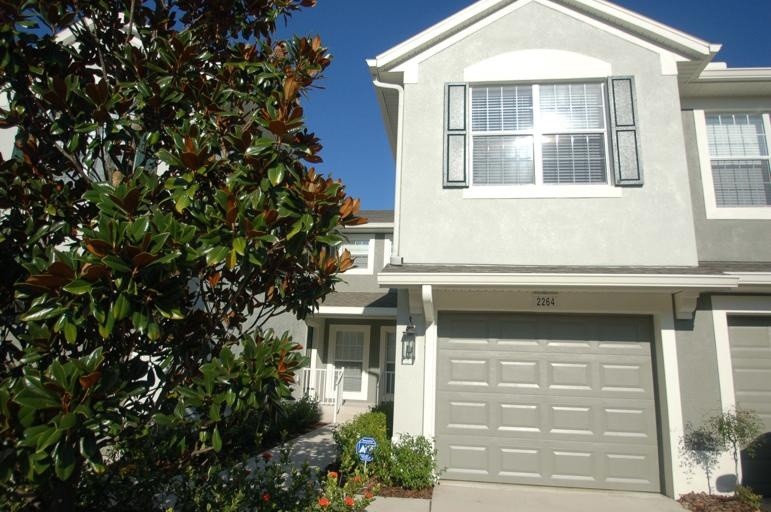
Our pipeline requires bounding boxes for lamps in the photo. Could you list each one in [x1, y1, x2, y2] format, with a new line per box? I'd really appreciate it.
[400, 318, 417, 363]
